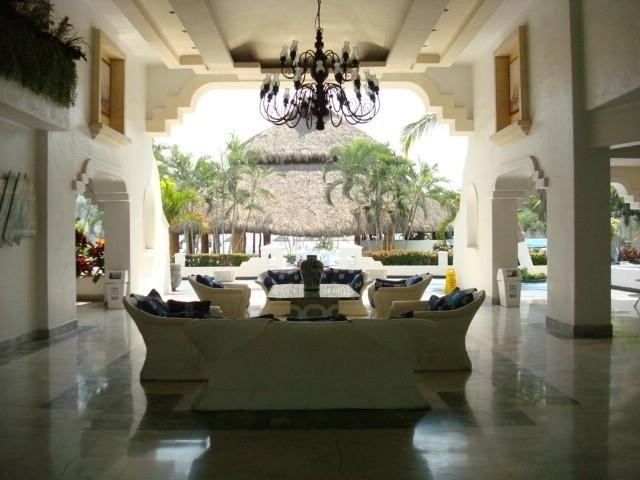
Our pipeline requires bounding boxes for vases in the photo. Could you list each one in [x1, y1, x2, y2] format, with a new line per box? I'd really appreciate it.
[301, 255, 323, 290]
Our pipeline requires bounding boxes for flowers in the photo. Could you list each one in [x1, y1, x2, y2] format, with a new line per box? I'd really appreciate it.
[75, 228, 104, 278]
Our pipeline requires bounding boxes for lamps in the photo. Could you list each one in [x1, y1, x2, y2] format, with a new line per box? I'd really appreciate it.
[258, 0, 380, 130]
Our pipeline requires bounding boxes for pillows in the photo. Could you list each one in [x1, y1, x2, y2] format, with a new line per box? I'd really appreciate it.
[197, 275, 214, 288]
[167, 299, 211, 318]
[160, 312, 209, 318]
[213, 283, 223, 288]
[443, 287, 474, 310]
[138, 289, 164, 316]
[426, 295, 449, 310]
[264, 268, 422, 291]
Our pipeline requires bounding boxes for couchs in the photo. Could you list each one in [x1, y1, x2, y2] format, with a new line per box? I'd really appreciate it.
[256, 269, 375, 315]
[182, 318, 435, 411]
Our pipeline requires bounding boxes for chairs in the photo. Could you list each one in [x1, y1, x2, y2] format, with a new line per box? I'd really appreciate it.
[122, 287, 222, 380]
[368, 272, 433, 314]
[391, 287, 485, 371]
[188, 274, 251, 317]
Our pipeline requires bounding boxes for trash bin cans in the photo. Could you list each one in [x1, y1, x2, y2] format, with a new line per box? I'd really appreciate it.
[103, 270, 129, 309]
[496, 267, 523, 307]
